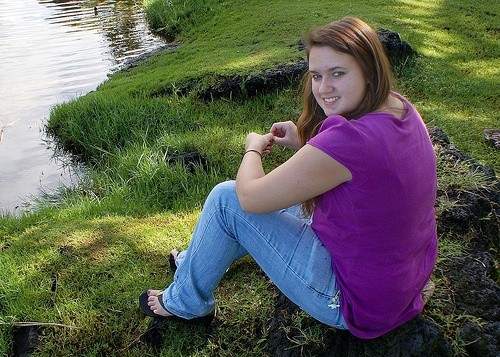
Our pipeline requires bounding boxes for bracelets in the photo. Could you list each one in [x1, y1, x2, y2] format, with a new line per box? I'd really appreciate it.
[244, 150, 262, 157]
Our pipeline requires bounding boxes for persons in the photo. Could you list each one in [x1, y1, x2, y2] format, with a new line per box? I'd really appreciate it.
[139, 15, 439, 340]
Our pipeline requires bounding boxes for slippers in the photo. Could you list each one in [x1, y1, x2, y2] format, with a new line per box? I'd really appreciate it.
[169, 250, 181, 272]
[139, 289, 215, 326]
